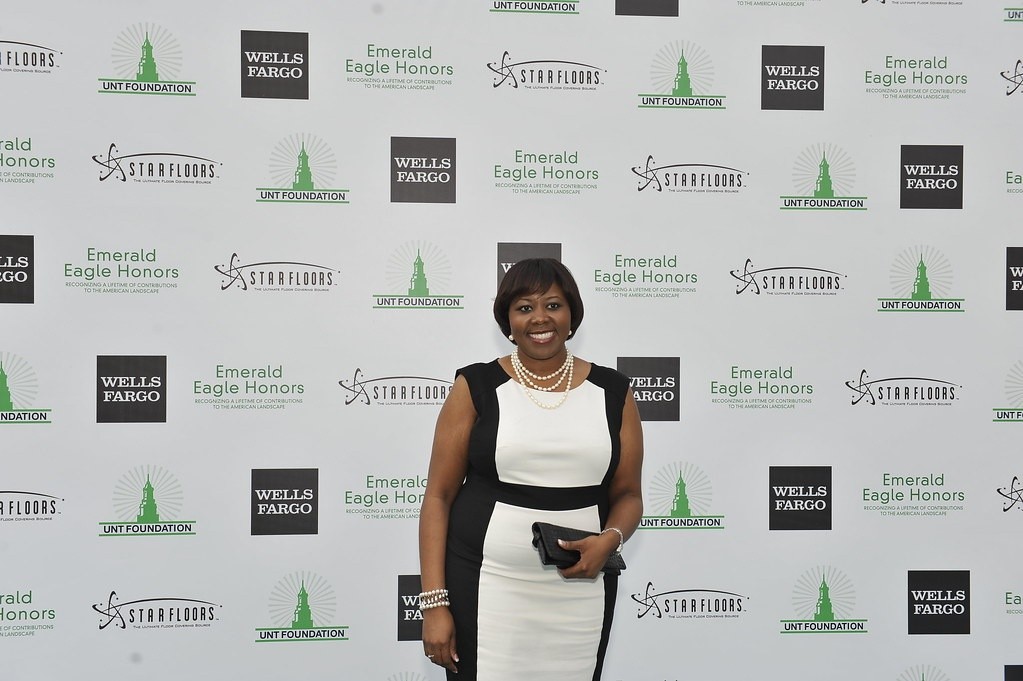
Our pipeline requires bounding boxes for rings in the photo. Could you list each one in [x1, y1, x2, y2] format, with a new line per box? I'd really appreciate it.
[428, 654, 434, 658]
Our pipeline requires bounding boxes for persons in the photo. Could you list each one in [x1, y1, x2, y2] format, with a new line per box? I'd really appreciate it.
[417, 257, 645, 681]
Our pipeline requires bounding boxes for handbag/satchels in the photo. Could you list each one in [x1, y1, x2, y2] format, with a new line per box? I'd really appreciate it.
[531, 522, 626, 577]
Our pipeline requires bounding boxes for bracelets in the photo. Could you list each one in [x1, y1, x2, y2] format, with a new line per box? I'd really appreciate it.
[418, 588, 451, 611]
[599, 527, 625, 555]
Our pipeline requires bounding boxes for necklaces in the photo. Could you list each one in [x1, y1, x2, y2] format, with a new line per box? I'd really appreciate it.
[510, 344, 573, 409]
